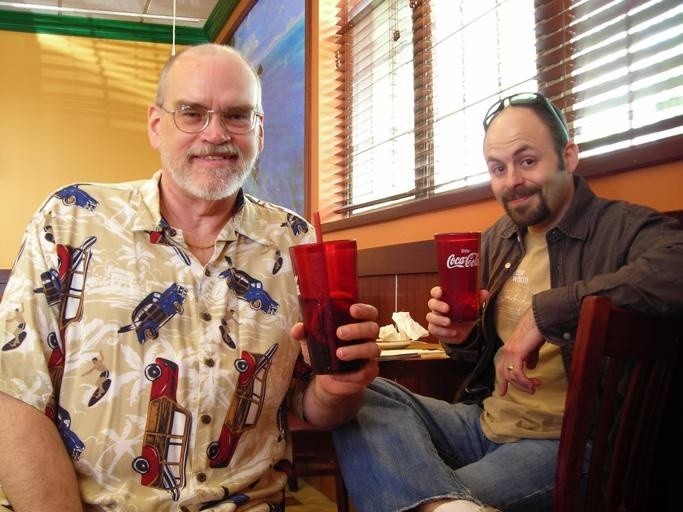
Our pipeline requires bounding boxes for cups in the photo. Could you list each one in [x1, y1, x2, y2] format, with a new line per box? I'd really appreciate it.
[434, 232, 483, 323]
[287, 239, 364, 376]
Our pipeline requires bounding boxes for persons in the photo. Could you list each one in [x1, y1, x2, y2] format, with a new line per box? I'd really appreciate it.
[331, 90, 681, 512]
[0, 41, 382, 511]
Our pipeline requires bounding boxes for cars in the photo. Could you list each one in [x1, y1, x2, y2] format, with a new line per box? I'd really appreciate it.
[206, 342, 279, 469]
[132, 357, 193, 501]
[118, 283, 189, 346]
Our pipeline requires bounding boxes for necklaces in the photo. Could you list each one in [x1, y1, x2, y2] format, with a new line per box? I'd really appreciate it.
[181, 236, 221, 253]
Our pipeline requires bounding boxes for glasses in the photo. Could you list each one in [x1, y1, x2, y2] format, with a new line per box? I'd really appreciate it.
[482, 91, 569, 145]
[160, 103, 263, 135]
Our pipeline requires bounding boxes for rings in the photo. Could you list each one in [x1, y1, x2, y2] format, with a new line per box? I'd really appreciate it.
[505, 366, 514, 370]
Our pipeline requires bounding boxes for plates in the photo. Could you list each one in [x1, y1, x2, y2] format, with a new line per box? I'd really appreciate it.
[375, 341, 413, 350]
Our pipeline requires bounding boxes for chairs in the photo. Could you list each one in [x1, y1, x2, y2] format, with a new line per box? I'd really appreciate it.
[285, 410, 350, 512]
[524, 294, 683, 512]
